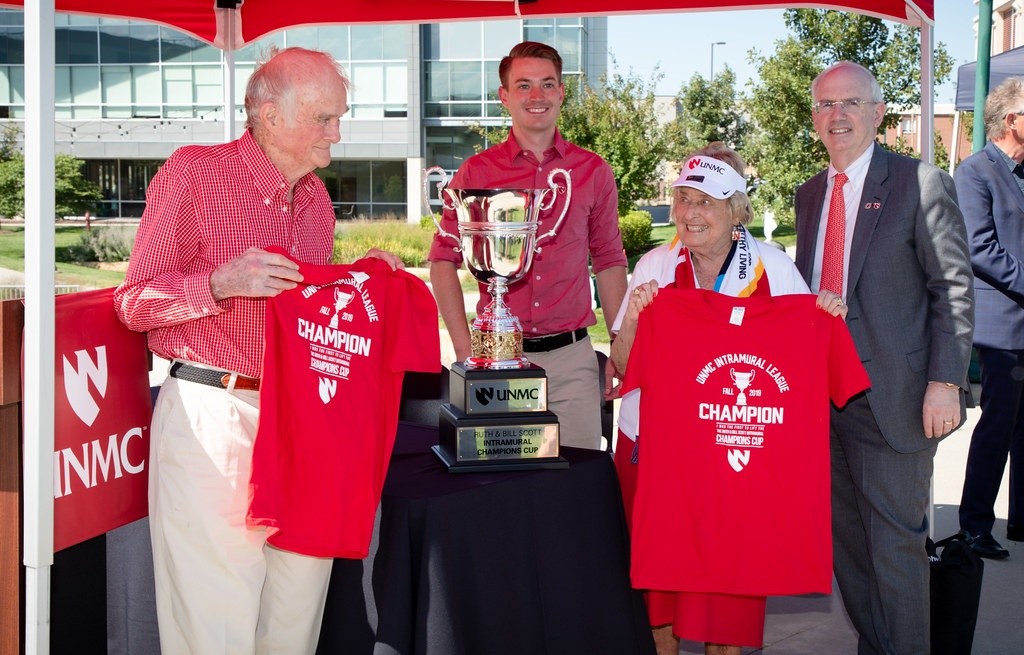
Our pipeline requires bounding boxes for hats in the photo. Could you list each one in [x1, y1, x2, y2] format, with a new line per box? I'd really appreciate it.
[670, 156, 746, 201]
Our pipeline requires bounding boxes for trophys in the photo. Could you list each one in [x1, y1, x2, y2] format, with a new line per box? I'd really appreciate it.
[423, 166, 569, 473]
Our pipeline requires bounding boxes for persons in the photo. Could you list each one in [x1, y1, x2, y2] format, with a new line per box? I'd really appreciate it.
[611, 141, 848, 655]
[794, 60, 975, 655]
[114, 48, 406, 655]
[953, 77, 1024, 559]
[429, 41, 629, 451]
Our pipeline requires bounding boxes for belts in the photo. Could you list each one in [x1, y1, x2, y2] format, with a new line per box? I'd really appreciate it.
[169, 359, 260, 392]
[523, 328, 588, 353]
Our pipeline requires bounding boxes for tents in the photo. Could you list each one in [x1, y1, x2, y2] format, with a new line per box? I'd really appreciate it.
[949, 45, 1024, 178]
[1, 0, 934, 655]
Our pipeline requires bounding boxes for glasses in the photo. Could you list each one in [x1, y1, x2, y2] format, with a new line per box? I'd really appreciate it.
[811, 101, 884, 114]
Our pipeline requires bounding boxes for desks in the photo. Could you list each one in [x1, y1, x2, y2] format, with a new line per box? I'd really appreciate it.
[358, 367, 658, 655]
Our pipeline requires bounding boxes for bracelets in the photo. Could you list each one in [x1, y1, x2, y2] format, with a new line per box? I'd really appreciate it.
[928, 380, 960, 389]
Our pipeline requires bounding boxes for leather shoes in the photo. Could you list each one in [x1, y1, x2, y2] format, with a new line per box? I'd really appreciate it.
[959, 525, 1024, 560]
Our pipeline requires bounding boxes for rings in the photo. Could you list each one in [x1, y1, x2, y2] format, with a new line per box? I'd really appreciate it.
[633, 288, 642, 295]
[943, 421, 952, 425]
[833, 297, 842, 306]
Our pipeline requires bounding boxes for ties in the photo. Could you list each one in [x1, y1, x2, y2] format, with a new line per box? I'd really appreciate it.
[819, 173, 849, 300]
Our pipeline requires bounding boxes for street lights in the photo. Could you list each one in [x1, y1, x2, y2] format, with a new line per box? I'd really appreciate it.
[711, 42, 727, 81]
